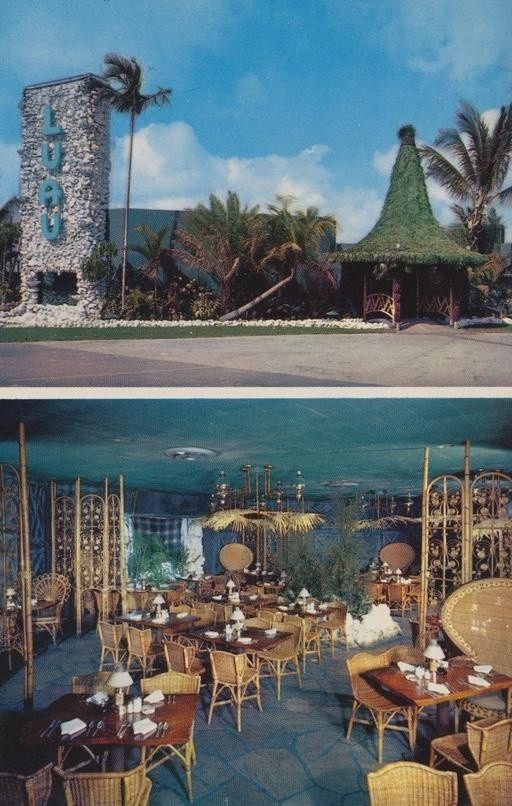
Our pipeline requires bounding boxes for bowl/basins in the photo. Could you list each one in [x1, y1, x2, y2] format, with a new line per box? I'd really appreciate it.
[142, 705, 155, 715]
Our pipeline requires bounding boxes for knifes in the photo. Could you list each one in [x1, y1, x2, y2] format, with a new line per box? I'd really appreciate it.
[420, 673, 495, 701]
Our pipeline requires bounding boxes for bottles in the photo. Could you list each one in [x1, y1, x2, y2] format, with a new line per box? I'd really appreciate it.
[127, 697, 142, 714]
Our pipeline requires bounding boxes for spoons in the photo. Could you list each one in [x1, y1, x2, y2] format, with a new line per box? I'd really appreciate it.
[167, 695, 176, 704]
[40, 718, 169, 740]
